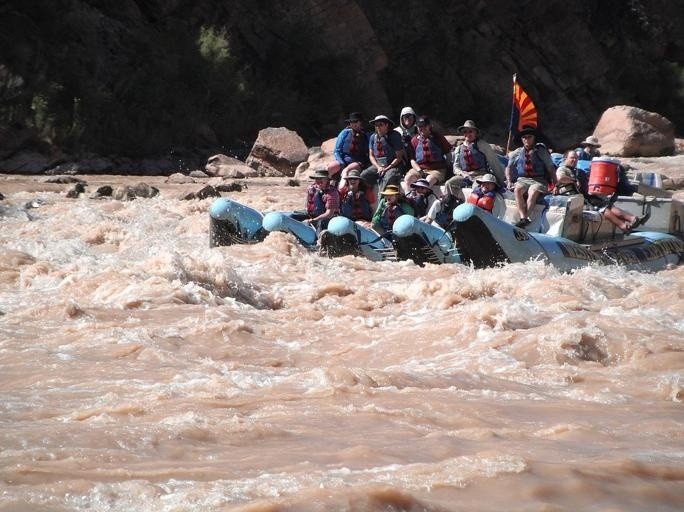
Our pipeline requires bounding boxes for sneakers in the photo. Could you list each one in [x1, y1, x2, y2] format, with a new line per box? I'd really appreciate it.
[624, 212, 649, 236]
[515, 218, 530, 227]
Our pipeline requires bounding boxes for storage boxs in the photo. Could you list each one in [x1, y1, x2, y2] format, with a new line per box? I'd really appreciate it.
[618, 194, 676, 234]
[496, 185, 617, 244]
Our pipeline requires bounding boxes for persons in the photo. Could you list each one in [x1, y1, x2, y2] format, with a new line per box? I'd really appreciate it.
[288, 107, 648, 243]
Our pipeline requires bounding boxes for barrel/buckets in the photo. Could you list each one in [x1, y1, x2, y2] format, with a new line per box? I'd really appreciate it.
[587, 155, 620, 197]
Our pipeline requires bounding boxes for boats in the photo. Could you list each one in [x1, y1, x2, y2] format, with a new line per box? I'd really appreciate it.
[494, 151, 662, 188]
[205, 170, 684, 269]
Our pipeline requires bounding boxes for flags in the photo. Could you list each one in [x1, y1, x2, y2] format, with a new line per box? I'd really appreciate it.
[511, 81, 539, 133]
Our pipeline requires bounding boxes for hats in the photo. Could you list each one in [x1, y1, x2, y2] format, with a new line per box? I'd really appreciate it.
[581, 136, 601, 148]
[518, 124, 541, 138]
[309, 169, 333, 180]
[457, 120, 480, 135]
[379, 185, 402, 196]
[476, 173, 502, 189]
[410, 178, 432, 190]
[343, 170, 366, 181]
[416, 115, 431, 127]
[344, 112, 395, 125]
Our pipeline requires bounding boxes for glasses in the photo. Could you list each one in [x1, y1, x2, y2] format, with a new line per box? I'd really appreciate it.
[403, 115, 415, 120]
